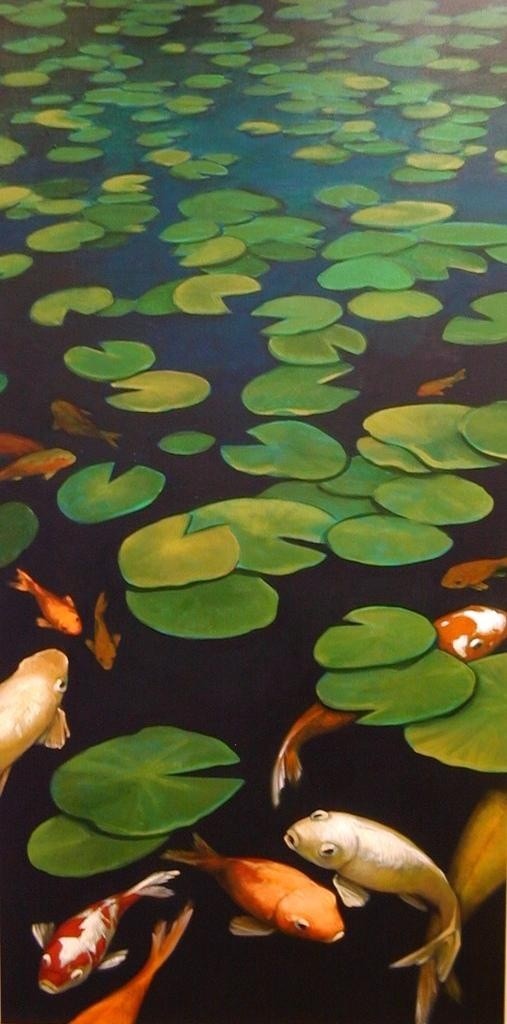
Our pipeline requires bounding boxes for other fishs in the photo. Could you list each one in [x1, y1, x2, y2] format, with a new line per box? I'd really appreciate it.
[159, 806, 465, 985]
